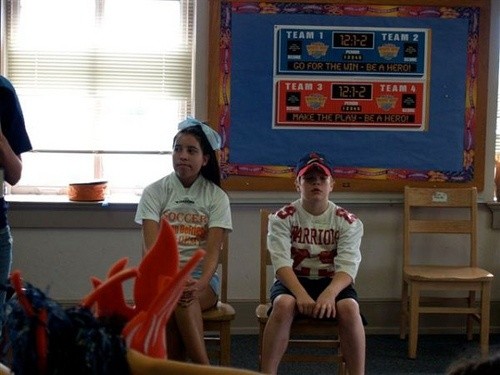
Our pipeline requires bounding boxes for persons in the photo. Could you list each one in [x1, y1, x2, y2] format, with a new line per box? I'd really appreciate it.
[256, 150, 369, 374]
[0, 75, 37, 375]
[134, 115, 234, 367]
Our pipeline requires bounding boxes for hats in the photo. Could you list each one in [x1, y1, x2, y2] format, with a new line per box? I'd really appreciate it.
[296, 152, 333, 177]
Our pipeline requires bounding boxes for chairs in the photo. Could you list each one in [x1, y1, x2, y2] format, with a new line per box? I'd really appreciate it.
[253, 208, 351, 375]
[141, 214, 237, 367]
[396, 185, 495, 360]
